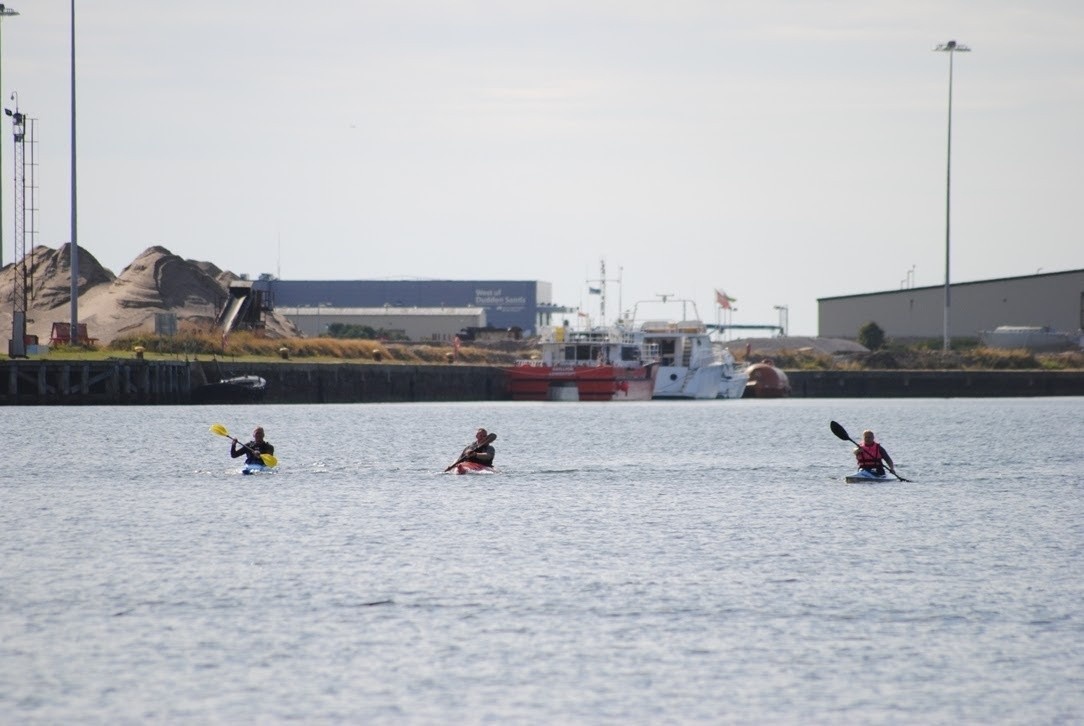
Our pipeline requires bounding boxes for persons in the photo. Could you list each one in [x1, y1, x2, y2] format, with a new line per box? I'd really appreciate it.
[853, 430, 894, 477]
[231, 426, 274, 472]
[457, 428, 495, 468]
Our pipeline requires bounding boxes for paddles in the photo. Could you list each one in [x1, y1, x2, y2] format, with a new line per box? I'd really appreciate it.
[444, 433, 498, 472]
[831, 420, 909, 482]
[210, 424, 277, 469]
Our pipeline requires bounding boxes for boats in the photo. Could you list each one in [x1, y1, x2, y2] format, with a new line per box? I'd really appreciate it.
[845, 474, 897, 483]
[541, 325, 749, 399]
[242, 464, 278, 475]
[456, 461, 494, 474]
[496, 358, 630, 401]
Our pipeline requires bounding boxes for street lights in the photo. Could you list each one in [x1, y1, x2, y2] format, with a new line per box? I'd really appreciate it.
[932, 39, 973, 351]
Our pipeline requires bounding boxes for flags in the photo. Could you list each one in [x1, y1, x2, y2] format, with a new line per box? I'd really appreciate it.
[712, 288, 737, 311]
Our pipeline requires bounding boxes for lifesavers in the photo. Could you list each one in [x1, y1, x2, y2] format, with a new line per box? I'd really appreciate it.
[454, 337, 460, 347]
